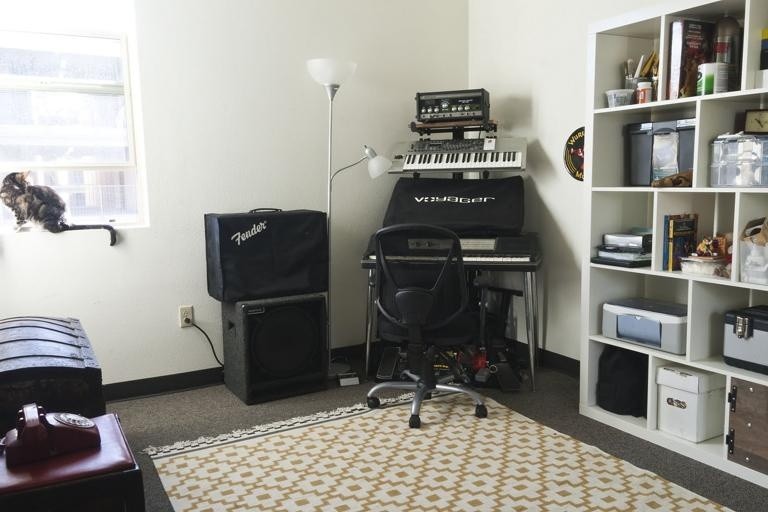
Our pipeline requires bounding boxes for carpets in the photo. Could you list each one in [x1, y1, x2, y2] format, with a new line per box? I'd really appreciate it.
[143, 380, 733, 512]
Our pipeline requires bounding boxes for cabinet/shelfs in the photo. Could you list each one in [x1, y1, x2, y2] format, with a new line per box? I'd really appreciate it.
[579, 0, 768, 492]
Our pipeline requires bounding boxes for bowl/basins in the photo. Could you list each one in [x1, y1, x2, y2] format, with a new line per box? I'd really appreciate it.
[606, 89, 634, 107]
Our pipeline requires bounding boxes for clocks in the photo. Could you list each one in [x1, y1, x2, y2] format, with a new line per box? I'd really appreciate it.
[743, 109, 768, 134]
[740, 240, 768, 286]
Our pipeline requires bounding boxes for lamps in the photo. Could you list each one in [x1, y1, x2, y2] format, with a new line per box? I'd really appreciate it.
[307, 57, 391, 377]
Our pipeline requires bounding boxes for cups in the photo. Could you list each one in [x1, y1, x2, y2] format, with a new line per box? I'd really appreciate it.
[624, 77, 647, 92]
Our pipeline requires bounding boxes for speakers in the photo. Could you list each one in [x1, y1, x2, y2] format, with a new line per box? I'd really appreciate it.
[203, 208, 328, 300]
[221, 294, 327, 403]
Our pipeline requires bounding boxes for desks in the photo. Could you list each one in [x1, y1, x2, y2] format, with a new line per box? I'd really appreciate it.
[0, 413, 144, 511]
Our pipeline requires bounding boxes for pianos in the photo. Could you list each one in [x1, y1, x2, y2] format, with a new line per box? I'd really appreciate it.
[389, 137, 527, 174]
[360, 234, 543, 272]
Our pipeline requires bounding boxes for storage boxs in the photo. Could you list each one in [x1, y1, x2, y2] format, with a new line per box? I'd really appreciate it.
[720, 303, 768, 375]
[727, 377, 768, 477]
[0, 316, 107, 441]
[623, 117, 696, 186]
[709, 137, 768, 188]
[655, 363, 726, 443]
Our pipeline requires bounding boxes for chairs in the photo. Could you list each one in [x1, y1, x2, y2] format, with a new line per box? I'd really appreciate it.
[366, 224, 487, 426]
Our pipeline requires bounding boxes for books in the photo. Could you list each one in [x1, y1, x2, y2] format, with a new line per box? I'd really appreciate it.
[590, 248, 651, 267]
[663, 213, 698, 271]
[666, 21, 717, 101]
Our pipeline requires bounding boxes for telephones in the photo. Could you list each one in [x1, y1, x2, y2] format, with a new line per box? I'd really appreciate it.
[0, 402, 100, 468]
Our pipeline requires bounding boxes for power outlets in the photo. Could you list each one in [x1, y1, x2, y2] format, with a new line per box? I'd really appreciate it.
[178, 305, 194, 328]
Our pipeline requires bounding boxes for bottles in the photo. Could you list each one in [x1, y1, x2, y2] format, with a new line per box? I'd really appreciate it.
[636, 82, 652, 103]
[715, 16, 742, 92]
[696, 63, 728, 95]
